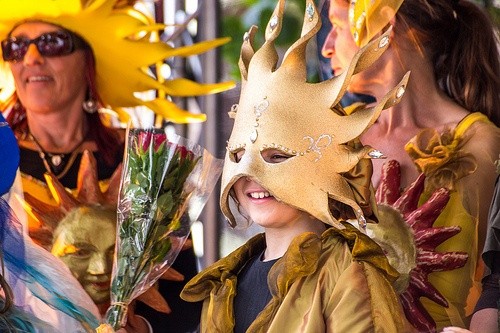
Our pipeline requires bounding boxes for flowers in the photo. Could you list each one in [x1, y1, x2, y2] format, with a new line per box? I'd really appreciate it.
[107, 123, 203, 330]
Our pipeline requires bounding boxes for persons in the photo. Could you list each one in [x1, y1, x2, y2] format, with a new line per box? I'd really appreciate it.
[0, 0, 201, 333]
[180, 82, 414, 333]
[321, 0, 500, 333]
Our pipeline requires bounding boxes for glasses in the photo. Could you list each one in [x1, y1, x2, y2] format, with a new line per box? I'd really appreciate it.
[1, 30, 88, 61]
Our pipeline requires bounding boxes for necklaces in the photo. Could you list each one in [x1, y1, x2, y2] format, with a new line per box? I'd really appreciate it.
[26, 131, 89, 178]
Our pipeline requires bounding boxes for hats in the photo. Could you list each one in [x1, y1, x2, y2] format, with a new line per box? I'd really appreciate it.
[0, 0, 237, 128]
[0, 112, 20, 198]
